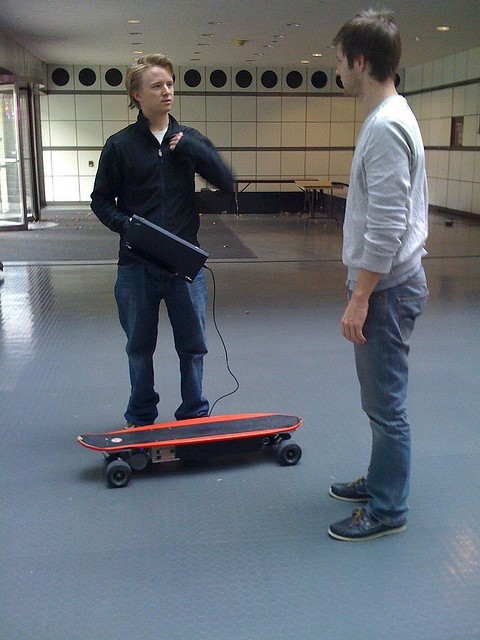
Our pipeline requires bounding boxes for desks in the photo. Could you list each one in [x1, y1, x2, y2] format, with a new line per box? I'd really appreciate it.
[233, 177, 319, 218]
[295, 179, 347, 225]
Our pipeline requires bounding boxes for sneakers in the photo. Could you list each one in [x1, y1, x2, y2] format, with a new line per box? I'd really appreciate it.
[328, 510, 408, 542]
[329, 476, 371, 502]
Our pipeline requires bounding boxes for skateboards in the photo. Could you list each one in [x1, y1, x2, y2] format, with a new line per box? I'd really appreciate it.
[77, 413, 303, 488]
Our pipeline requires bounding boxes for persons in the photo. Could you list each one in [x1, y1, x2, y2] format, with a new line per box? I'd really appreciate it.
[327, 9, 427, 544]
[90, 54, 234, 430]
